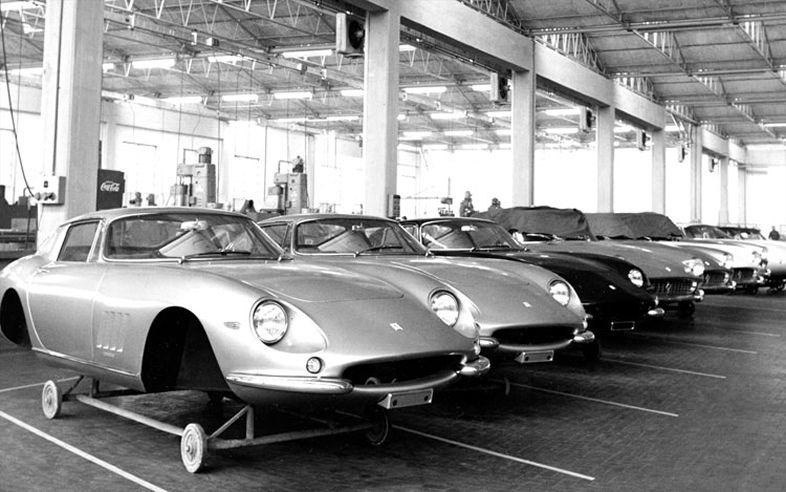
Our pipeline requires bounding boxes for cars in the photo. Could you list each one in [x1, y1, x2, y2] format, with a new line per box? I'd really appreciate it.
[584, 213, 786, 292]
[228, 214, 594, 372]
[474, 208, 705, 316]
[0, 207, 491, 408]
[367, 217, 666, 333]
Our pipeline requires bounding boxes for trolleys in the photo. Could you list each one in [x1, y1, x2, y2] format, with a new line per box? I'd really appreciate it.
[41, 372, 393, 473]
